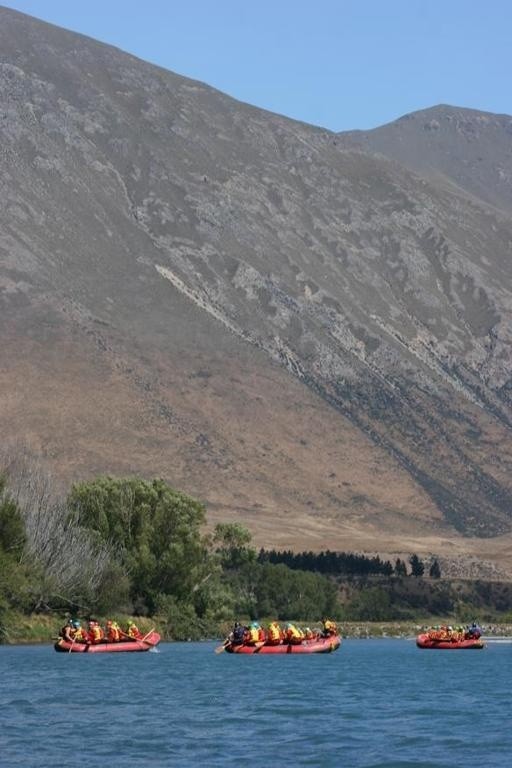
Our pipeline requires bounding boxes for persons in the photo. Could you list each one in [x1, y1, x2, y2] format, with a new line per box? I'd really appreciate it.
[62, 616, 144, 645]
[420, 622, 481, 643]
[230, 616, 339, 647]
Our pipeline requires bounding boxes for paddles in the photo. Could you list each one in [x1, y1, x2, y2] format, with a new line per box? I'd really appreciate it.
[119, 632, 155, 647]
[253, 640, 268, 653]
[214, 642, 232, 654]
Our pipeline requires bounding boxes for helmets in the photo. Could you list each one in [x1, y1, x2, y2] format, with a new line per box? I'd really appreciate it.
[234, 619, 294, 629]
[431, 622, 479, 631]
[66, 617, 136, 628]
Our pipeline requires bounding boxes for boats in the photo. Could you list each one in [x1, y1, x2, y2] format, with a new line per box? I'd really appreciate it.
[54, 631, 161, 652]
[416, 633, 484, 648]
[225, 633, 341, 654]
